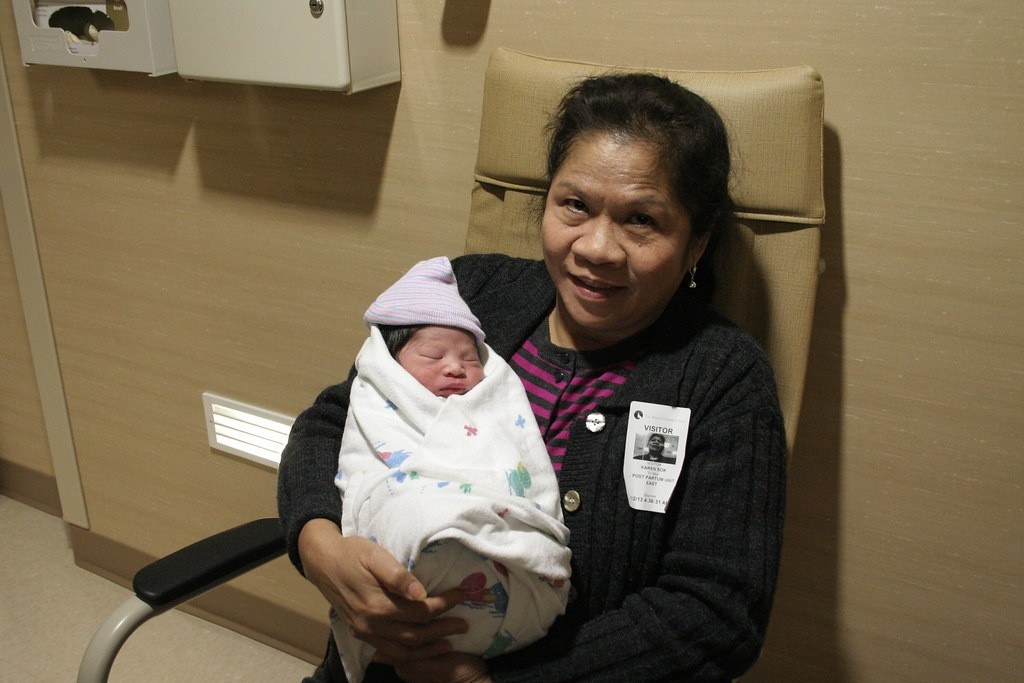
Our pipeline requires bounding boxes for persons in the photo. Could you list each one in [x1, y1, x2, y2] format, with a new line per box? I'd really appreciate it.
[276, 72, 791, 683]
[633, 433, 676, 465]
[331, 251, 572, 682]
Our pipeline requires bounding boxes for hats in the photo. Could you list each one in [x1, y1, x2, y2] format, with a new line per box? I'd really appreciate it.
[364, 256, 486, 347]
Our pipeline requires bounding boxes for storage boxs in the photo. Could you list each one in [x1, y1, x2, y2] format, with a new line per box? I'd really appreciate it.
[168, 0, 402, 95]
[11, 0, 178, 77]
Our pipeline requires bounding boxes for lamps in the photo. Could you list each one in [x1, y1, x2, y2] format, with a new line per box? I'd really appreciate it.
[201, 392, 296, 470]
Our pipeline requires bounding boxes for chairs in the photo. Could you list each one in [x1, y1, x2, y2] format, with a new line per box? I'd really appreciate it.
[78, 44, 826, 682]
